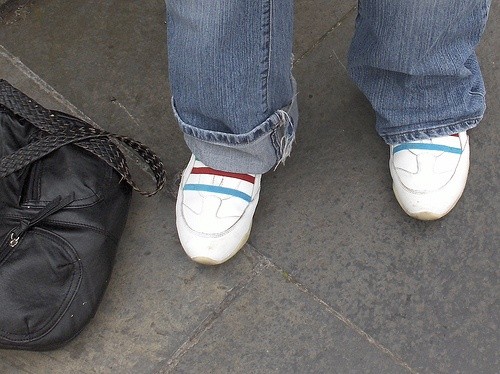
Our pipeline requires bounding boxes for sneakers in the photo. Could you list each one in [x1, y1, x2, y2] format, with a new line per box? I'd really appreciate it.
[385, 124, 473, 226]
[174, 148, 267, 268]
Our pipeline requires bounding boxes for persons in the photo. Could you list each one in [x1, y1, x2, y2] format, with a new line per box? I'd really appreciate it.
[162, 0, 494, 267]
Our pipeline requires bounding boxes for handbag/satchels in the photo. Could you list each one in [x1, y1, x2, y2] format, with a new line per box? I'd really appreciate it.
[0, 82, 168, 359]
